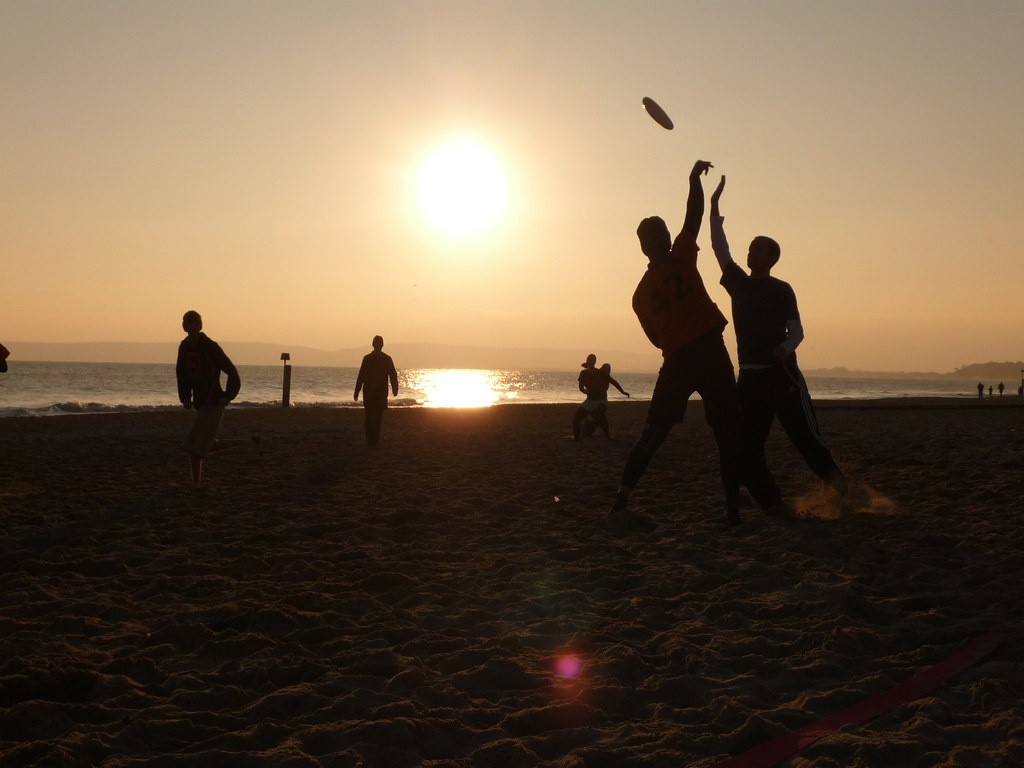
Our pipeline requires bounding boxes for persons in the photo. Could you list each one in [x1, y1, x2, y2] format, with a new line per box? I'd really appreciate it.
[354, 336, 398, 448]
[977, 382, 1004, 398]
[168, 311, 241, 509]
[570, 354, 630, 442]
[608, 161, 787, 516]
[710, 175, 847, 529]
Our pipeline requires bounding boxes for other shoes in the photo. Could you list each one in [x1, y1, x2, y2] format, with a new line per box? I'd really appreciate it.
[609, 501, 625, 515]
[726, 511, 741, 527]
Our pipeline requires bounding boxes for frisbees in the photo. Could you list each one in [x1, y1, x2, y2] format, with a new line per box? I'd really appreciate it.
[642, 96, 675, 130]
